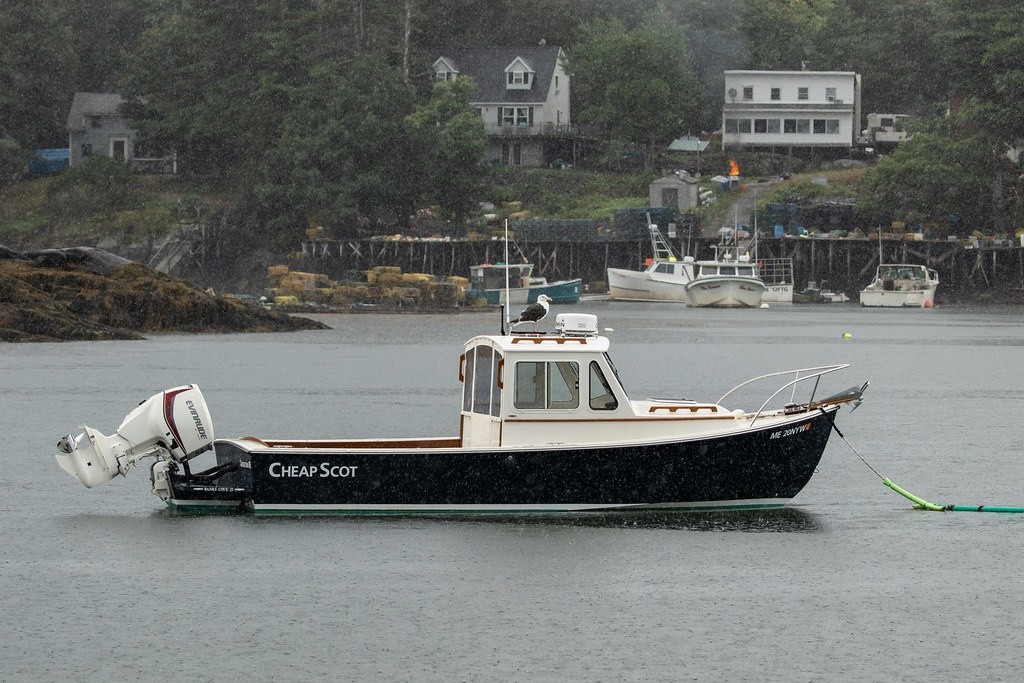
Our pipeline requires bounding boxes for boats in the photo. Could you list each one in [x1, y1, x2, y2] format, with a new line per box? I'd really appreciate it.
[462, 263, 585, 308]
[54, 220, 869, 520]
[607, 198, 850, 308]
[859, 224, 939, 308]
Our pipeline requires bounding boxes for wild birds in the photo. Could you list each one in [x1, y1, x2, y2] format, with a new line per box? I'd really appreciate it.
[508, 294, 553, 333]
